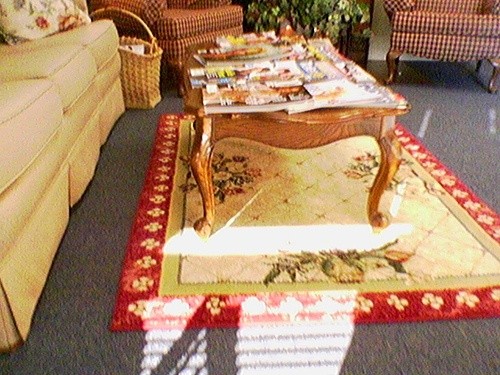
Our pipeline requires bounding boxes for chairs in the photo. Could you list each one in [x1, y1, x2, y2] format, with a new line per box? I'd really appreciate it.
[381, 0, 500, 94]
[87, 0, 244, 98]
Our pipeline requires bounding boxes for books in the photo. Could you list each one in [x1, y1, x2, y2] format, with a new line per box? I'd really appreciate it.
[186, 31, 408, 116]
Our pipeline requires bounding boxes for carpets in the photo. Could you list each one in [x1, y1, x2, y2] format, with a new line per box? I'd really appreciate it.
[107, 113, 500, 333]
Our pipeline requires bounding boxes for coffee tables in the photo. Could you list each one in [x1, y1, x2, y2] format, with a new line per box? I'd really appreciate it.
[178, 34, 412, 240]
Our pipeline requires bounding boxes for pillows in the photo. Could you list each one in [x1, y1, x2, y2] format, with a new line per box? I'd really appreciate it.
[0, 0, 92, 46]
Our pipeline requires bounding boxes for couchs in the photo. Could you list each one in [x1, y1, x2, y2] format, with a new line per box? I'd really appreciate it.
[0, 19, 126, 355]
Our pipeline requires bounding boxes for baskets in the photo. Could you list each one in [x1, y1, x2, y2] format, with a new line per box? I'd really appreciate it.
[88, 6, 162, 111]
